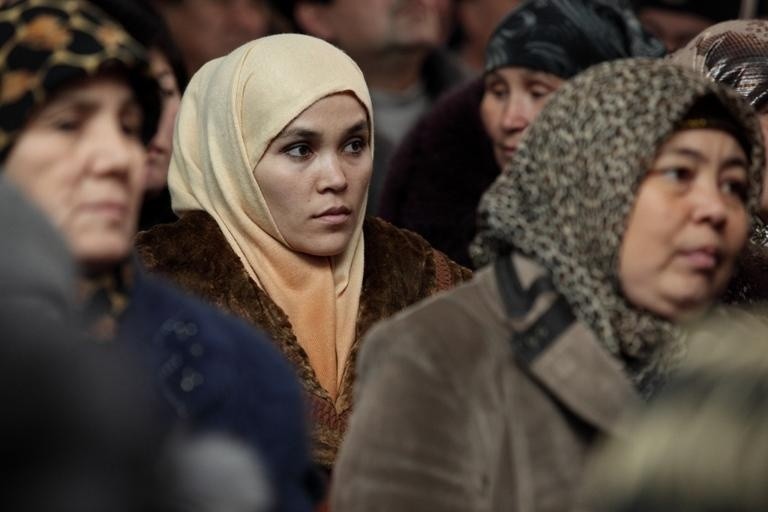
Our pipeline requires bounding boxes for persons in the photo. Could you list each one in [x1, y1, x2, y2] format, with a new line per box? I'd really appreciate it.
[292, 1, 473, 181]
[450, 1, 524, 85]
[323, 21, 768, 512]
[0, 1, 318, 511]
[376, 2, 670, 268]
[635, 1, 745, 51]
[131, 34, 474, 468]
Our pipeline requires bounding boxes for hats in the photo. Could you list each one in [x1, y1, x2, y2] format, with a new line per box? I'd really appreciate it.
[482, 0, 631, 82]
